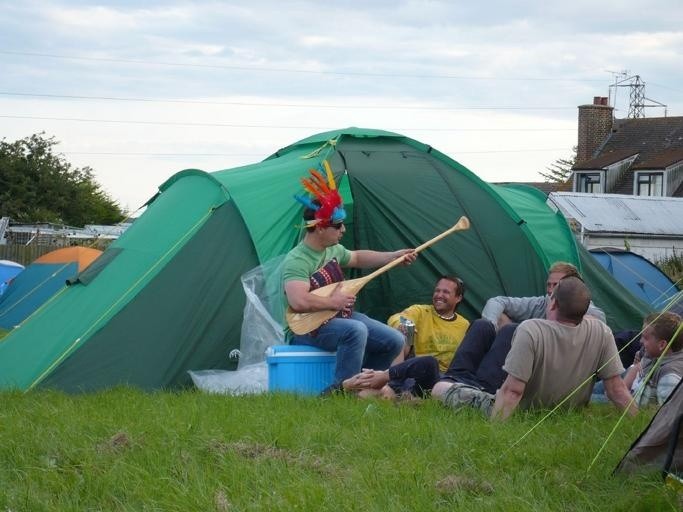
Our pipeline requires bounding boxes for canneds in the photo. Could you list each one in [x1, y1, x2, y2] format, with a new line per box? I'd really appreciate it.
[404, 323, 414, 344]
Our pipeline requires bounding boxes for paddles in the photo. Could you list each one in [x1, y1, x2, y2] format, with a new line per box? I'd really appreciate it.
[284, 214, 471, 336]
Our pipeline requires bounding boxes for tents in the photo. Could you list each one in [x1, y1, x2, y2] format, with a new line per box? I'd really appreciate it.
[0, 259, 24, 295]
[587, 246, 682, 320]
[0, 127, 649, 396]
[609, 370, 683, 491]
[0, 247, 102, 330]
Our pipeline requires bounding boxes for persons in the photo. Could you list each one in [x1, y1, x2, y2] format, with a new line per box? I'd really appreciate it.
[280, 198, 418, 394]
[430, 273, 641, 422]
[387, 273, 471, 374]
[580, 310, 683, 405]
[319, 318, 519, 405]
[480, 261, 606, 331]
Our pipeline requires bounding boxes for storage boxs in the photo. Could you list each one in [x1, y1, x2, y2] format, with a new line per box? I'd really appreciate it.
[265, 343, 337, 398]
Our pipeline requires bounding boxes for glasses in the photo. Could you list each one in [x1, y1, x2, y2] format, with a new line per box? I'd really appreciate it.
[326, 222, 342, 230]
